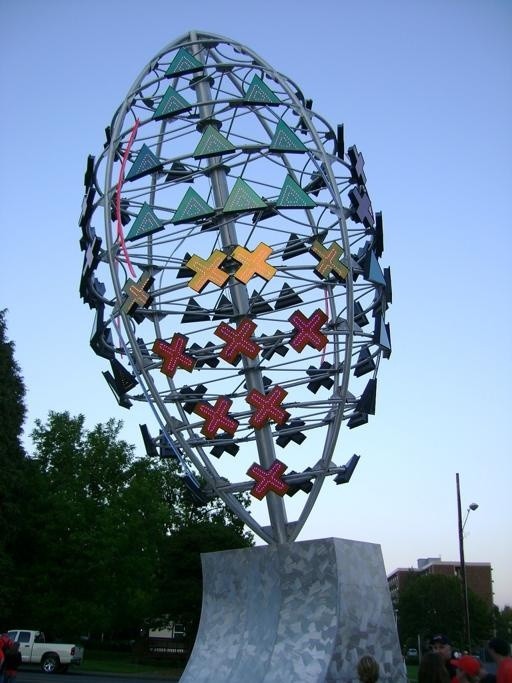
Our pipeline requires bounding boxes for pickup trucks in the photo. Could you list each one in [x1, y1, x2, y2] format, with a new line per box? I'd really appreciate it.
[0, 628, 85, 671]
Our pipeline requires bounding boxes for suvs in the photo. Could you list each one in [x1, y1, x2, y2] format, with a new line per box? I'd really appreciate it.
[407, 647, 418, 657]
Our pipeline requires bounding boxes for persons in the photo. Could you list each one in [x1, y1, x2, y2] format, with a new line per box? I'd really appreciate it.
[357, 655, 379, 683]
[417, 653, 450, 682]
[448, 654, 481, 683]
[0, 633, 14, 683]
[428, 632, 456, 679]
[2, 642, 23, 683]
[487, 637, 512, 683]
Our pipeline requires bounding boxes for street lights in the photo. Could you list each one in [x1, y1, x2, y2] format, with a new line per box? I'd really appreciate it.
[454, 472, 482, 656]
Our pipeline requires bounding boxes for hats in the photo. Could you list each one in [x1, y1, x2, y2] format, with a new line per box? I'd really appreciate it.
[429, 634, 454, 646]
[450, 655, 480, 677]
